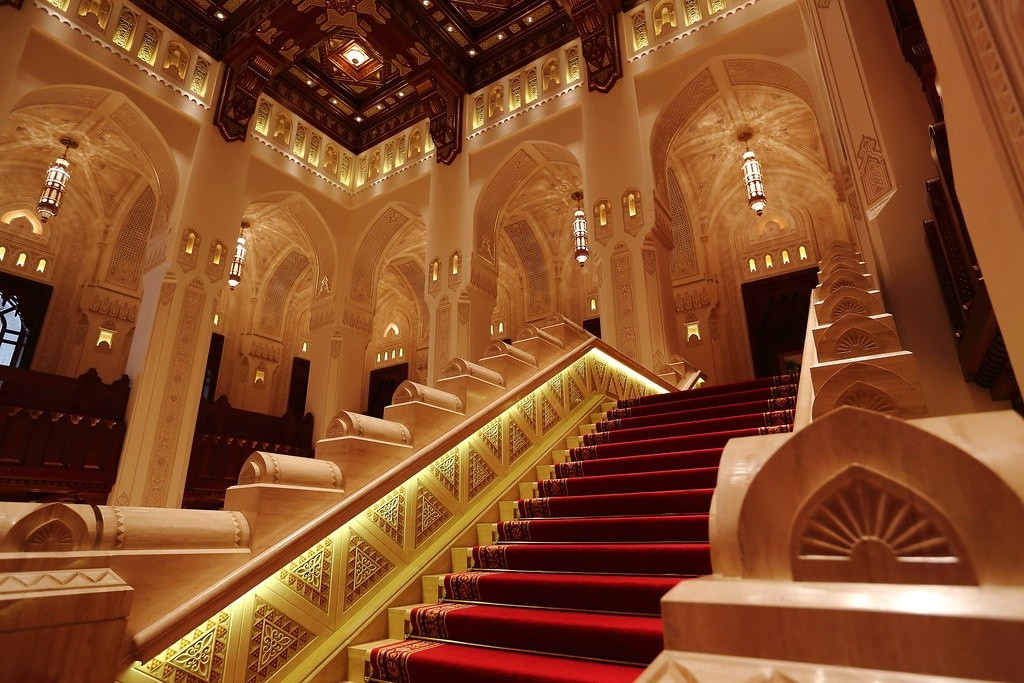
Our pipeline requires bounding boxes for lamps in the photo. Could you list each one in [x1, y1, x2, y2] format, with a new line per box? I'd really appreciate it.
[346, 47, 367, 67]
[227, 219, 251, 291]
[737, 131, 769, 216]
[570, 191, 591, 268]
[35, 133, 79, 223]
